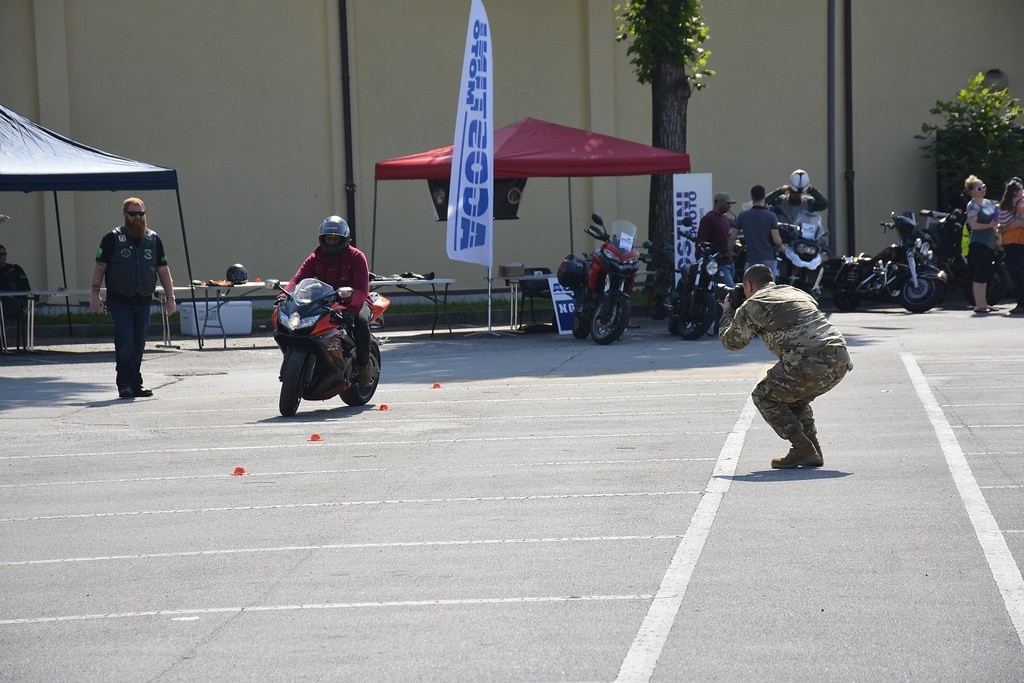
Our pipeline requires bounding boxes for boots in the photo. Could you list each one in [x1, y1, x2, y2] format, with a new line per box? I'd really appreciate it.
[771, 431, 820, 468]
[807, 433, 823, 466]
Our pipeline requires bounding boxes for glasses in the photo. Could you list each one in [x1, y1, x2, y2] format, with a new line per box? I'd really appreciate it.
[0, 252, 7, 256]
[125, 210, 145, 216]
[973, 184, 985, 191]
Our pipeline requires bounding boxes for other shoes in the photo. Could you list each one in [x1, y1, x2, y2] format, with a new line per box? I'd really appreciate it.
[358, 358, 372, 379]
[119, 384, 136, 396]
[137, 384, 153, 396]
[988, 305, 999, 310]
[1009, 306, 1024, 314]
[974, 307, 991, 312]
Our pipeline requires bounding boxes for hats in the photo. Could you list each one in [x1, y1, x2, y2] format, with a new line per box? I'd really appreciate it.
[714, 191, 736, 204]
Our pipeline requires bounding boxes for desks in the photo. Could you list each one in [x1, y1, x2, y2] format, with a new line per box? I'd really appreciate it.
[485, 270, 657, 333]
[0, 285, 195, 353]
[192, 278, 458, 349]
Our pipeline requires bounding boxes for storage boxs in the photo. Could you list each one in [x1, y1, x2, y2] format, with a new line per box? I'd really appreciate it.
[499, 265, 525, 277]
[557, 255, 585, 287]
[180, 302, 252, 335]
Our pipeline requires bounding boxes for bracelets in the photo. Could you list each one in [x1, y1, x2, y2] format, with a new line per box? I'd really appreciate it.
[91, 285, 100, 292]
[167, 296, 175, 300]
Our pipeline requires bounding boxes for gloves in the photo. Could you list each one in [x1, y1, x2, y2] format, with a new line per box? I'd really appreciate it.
[274, 296, 285, 305]
[344, 311, 355, 331]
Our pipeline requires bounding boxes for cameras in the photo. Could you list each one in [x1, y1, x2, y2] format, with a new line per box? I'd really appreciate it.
[714, 283, 746, 310]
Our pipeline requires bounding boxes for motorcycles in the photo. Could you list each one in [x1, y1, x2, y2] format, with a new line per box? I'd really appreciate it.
[732, 215, 831, 315]
[556, 213, 654, 344]
[668, 216, 728, 340]
[824, 210, 1012, 315]
[264, 278, 390, 418]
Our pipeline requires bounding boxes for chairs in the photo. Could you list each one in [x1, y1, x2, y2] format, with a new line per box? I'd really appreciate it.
[519, 266, 557, 332]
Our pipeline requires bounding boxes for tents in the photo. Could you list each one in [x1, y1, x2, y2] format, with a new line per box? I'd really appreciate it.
[0, 103, 202, 351]
[371, 117, 692, 272]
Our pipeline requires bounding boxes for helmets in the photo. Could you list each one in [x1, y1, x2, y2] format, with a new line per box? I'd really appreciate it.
[790, 169, 810, 193]
[227, 264, 248, 284]
[319, 216, 350, 252]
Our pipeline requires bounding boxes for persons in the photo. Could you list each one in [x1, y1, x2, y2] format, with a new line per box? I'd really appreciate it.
[998, 177, 1024, 314]
[89, 197, 176, 397]
[764, 185, 828, 225]
[698, 192, 736, 288]
[727, 184, 782, 279]
[718, 264, 853, 468]
[965, 175, 1000, 312]
[273, 215, 374, 390]
[0, 245, 30, 318]
